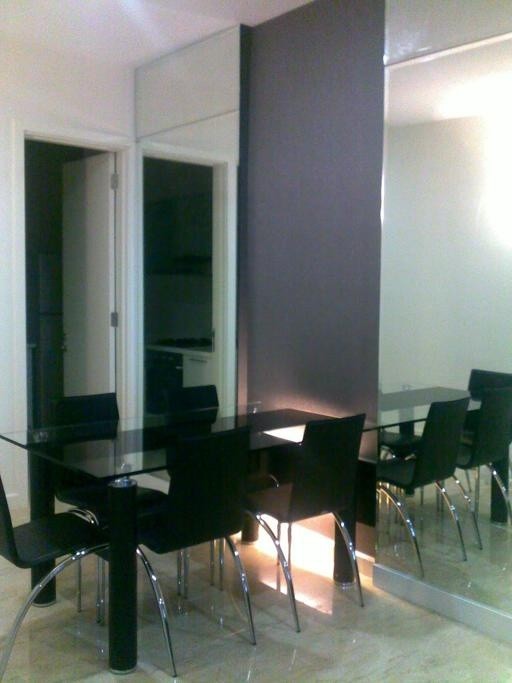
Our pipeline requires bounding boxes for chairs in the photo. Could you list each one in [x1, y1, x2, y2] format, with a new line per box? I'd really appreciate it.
[374, 368, 512, 582]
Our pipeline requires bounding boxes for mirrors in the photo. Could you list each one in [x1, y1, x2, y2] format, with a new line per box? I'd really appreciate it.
[129, 21, 242, 482]
[375, 0, 512, 614]
